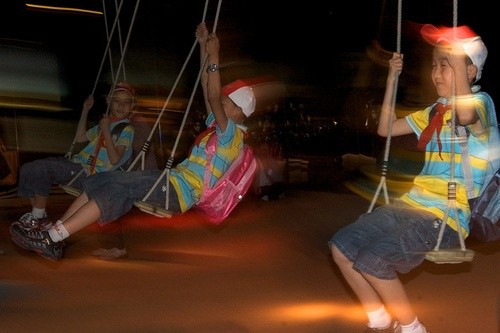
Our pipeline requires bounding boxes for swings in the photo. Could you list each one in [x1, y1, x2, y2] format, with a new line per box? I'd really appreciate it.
[361, 0, 475, 264]
[126, 0, 222, 218]
[58, 0, 139, 200]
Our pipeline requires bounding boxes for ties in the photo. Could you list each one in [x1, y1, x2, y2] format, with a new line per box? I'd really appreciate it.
[100, 113, 128, 141]
[190, 124, 215, 157]
[418, 100, 452, 163]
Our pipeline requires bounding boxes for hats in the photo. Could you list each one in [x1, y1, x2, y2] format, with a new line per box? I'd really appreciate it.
[420, 23, 488, 72]
[219, 80, 260, 118]
[110, 80, 136, 97]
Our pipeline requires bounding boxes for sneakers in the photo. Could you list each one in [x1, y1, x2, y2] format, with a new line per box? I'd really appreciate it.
[8, 224, 65, 259]
[11, 230, 62, 263]
[11, 212, 52, 231]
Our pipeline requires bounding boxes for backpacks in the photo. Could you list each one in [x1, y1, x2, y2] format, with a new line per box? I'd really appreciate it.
[467, 167, 500, 244]
[196, 120, 258, 227]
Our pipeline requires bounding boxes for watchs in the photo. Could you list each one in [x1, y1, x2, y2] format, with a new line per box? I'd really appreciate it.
[208, 64, 218, 72]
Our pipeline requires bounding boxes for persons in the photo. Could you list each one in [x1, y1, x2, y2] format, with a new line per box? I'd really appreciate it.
[327, 23, 498, 333]
[9, 21, 256, 263]
[12, 82, 135, 231]
